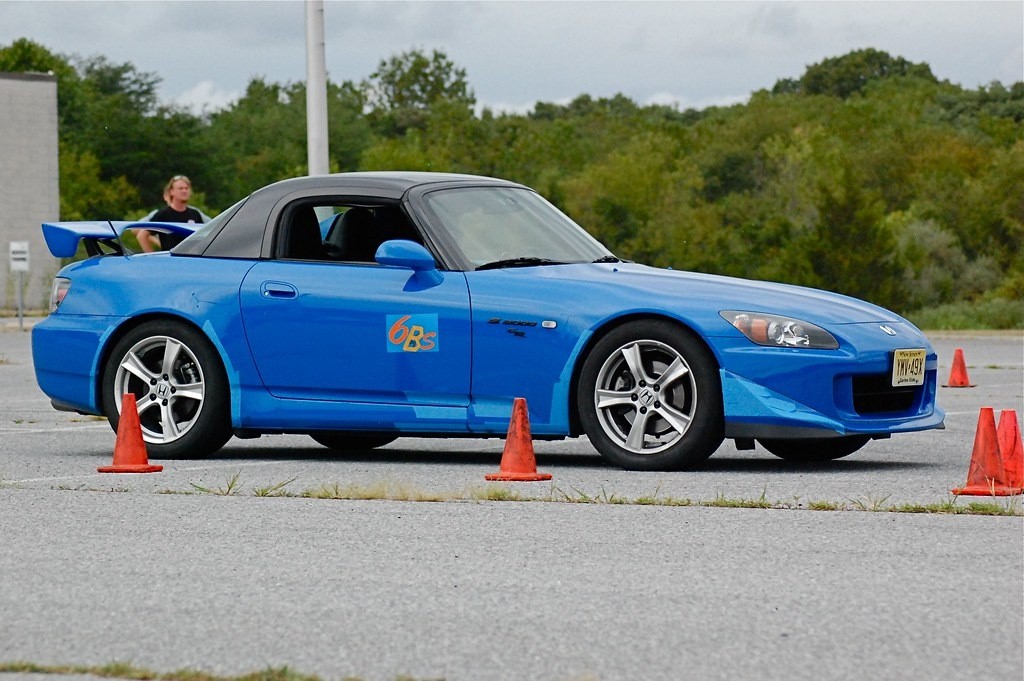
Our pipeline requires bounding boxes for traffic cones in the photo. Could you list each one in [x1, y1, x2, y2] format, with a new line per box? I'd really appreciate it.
[95, 392, 164, 473]
[951, 407, 1024, 497]
[485, 397, 552, 483]
[941, 348, 976, 388]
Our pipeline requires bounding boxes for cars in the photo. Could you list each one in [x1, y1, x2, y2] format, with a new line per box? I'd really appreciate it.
[28, 170, 945, 473]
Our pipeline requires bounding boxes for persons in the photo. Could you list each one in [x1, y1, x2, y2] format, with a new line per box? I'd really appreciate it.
[132, 175, 213, 254]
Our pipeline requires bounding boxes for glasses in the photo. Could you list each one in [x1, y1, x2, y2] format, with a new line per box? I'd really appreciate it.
[173, 175, 188, 181]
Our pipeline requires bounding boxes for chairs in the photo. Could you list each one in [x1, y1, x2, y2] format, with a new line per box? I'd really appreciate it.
[327, 208, 376, 263]
[285, 205, 327, 262]
